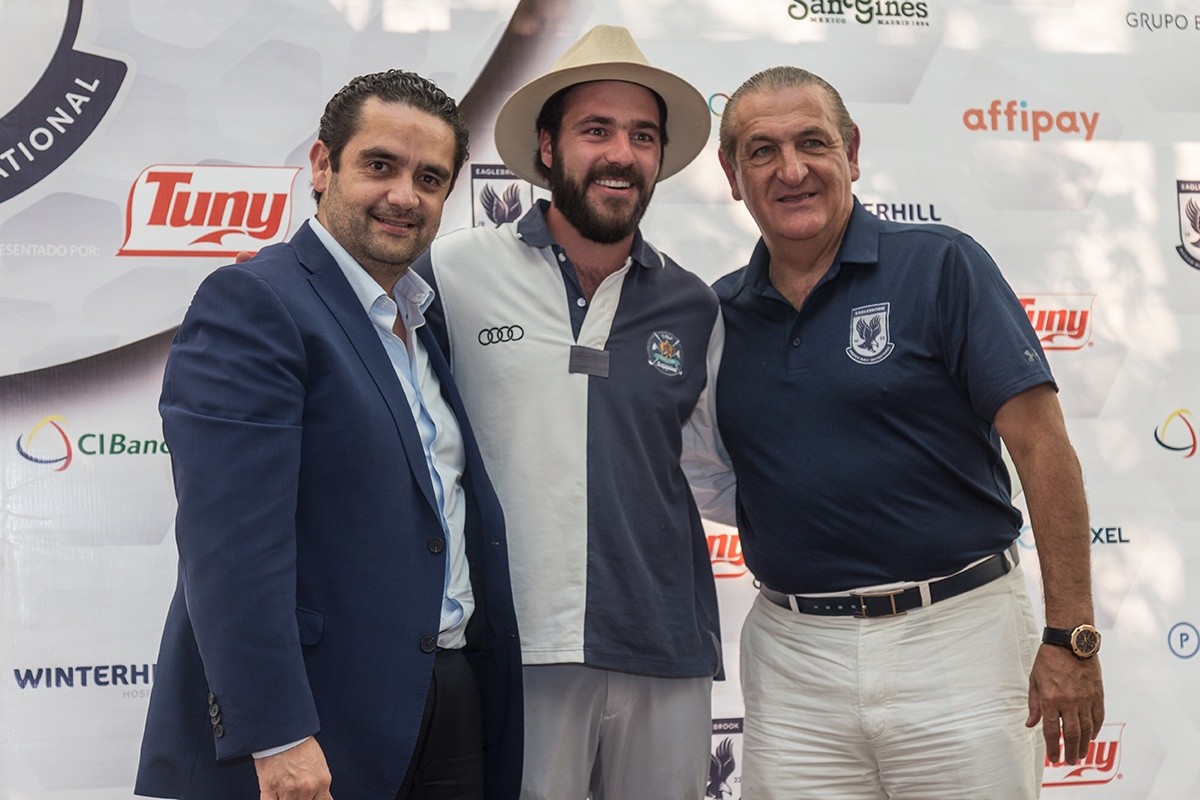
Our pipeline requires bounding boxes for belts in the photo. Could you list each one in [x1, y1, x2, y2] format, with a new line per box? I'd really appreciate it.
[758, 541, 1021, 620]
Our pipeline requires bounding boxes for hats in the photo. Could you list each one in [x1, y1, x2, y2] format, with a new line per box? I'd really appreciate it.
[492, 24, 711, 187]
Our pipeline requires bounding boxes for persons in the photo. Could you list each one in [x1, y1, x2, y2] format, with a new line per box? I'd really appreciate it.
[709, 65, 1104, 800]
[408, 28, 742, 800]
[129, 65, 526, 799]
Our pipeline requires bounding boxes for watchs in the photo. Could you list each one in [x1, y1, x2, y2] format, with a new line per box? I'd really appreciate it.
[1042, 625, 1101, 659]
